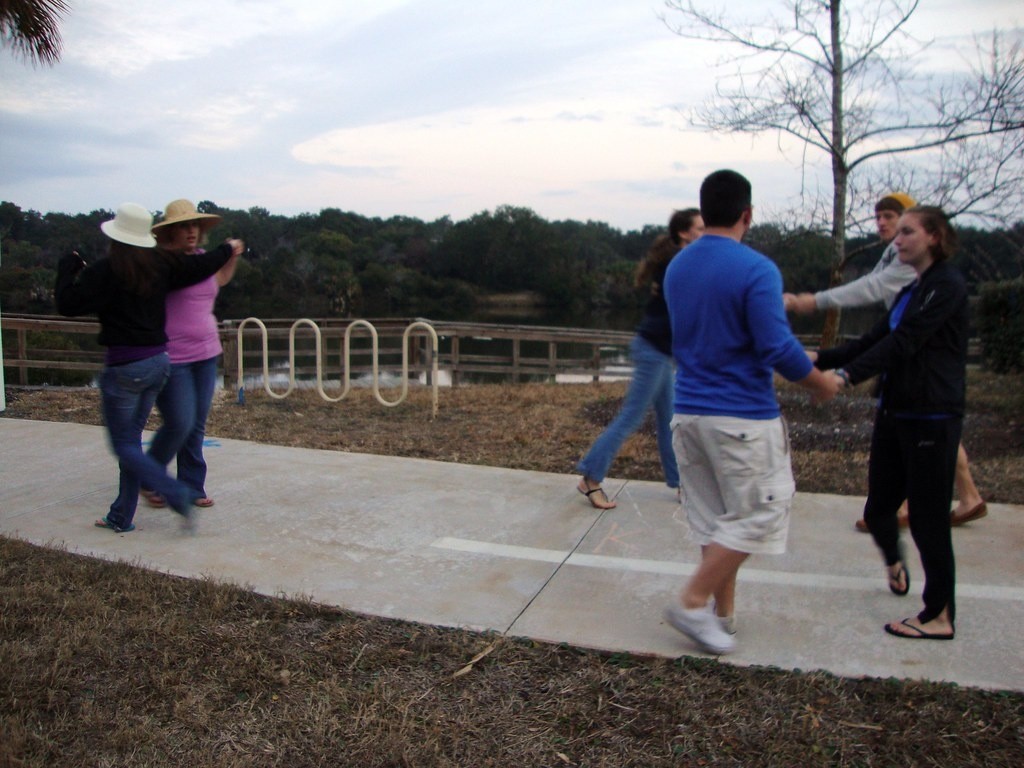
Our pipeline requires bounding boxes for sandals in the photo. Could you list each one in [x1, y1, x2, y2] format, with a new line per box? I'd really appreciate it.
[577, 478, 617, 510]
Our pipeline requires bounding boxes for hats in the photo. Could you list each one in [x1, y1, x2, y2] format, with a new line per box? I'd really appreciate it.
[874, 192, 917, 212]
[150, 199, 221, 240]
[100, 202, 158, 249]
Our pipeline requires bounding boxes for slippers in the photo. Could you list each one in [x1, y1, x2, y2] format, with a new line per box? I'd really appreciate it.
[142, 491, 165, 508]
[884, 616, 955, 641]
[886, 542, 911, 597]
[94, 516, 136, 532]
[195, 497, 215, 507]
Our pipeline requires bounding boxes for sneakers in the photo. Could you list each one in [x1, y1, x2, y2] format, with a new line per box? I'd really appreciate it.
[711, 599, 738, 635]
[660, 588, 735, 655]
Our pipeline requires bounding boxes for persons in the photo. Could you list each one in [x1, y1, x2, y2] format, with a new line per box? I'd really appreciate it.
[663, 169, 837, 654]
[577, 207, 799, 508]
[800, 205, 969, 639]
[56, 204, 243, 531]
[795, 192, 987, 532]
[139, 200, 243, 509]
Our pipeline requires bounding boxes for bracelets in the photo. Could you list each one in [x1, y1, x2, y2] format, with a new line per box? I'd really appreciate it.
[835, 368, 847, 383]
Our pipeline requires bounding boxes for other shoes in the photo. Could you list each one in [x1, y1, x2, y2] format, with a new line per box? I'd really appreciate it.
[950, 501, 989, 526]
[856, 514, 908, 533]
